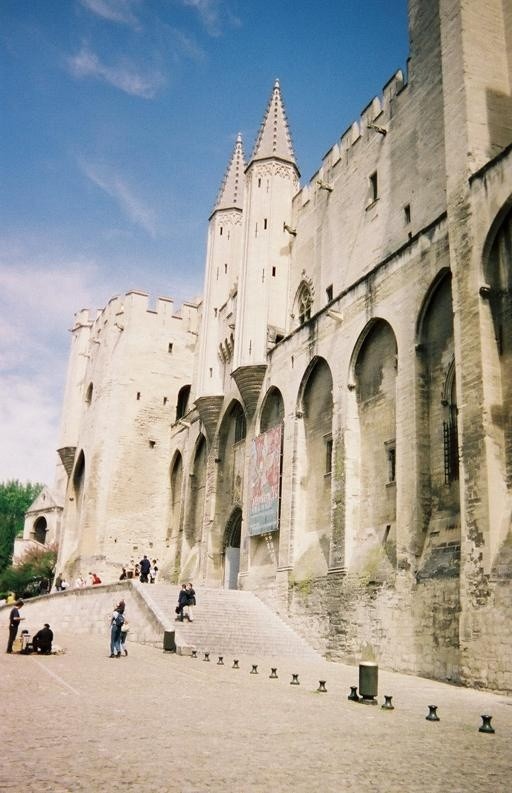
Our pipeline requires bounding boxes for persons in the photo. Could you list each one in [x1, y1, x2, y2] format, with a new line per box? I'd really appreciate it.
[113, 607, 130, 656]
[108, 598, 126, 658]
[178, 583, 188, 621]
[182, 582, 197, 621]
[31, 622, 54, 655]
[0, 553, 158, 610]
[5, 599, 25, 654]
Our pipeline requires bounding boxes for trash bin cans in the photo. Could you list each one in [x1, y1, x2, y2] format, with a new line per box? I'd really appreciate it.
[163, 629, 175, 654]
[358, 661, 379, 705]
